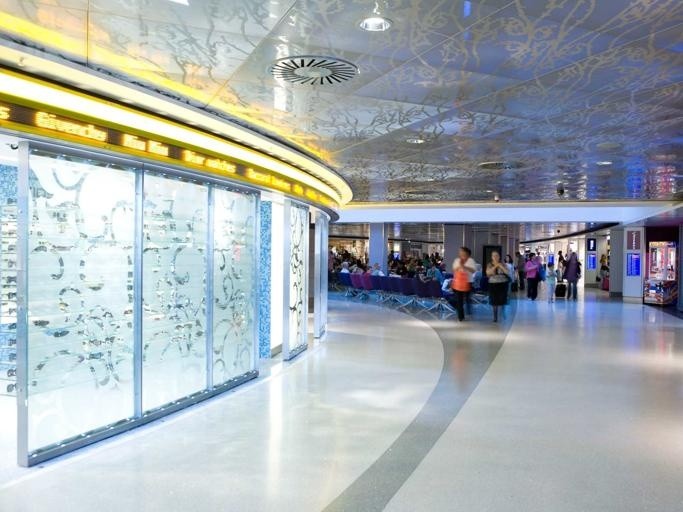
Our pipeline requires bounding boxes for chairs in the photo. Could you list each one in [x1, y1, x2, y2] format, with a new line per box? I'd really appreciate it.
[329, 270, 490, 321]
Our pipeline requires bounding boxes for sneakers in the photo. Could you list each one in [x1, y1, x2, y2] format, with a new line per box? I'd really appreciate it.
[547, 297, 554, 302]
[459, 316, 466, 321]
[573, 296, 576, 300]
[494, 316, 498, 322]
[567, 295, 571, 299]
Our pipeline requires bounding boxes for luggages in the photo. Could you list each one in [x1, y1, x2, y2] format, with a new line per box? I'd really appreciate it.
[603, 272, 609, 291]
[555, 284, 567, 298]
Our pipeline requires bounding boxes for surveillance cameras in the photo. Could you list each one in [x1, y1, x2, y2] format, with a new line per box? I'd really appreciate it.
[556, 189, 564, 196]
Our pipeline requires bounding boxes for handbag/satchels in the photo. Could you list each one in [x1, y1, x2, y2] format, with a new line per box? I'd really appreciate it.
[451, 267, 470, 292]
[535, 270, 542, 283]
[503, 272, 513, 282]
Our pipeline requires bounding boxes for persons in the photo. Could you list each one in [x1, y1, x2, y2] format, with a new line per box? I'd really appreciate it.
[598, 254, 609, 290]
[471, 264, 483, 292]
[486, 250, 511, 323]
[328, 244, 454, 294]
[452, 246, 477, 322]
[504, 250, 581, 301]
